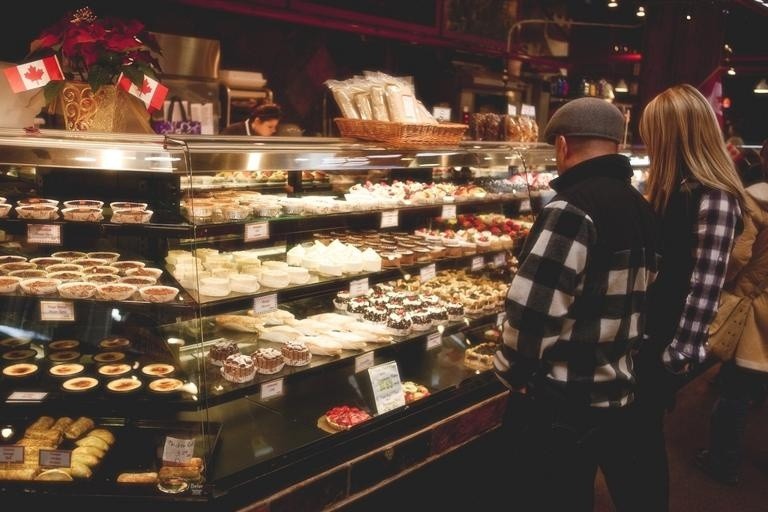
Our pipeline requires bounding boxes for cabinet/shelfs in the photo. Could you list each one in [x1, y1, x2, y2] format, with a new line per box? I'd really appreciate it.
[0, 152, 662, 501]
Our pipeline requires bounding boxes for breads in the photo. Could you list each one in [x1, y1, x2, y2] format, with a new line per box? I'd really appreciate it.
[471, 114, 539, 143]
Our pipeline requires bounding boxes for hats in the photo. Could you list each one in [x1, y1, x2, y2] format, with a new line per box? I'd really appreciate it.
[543, 96, 626, 144]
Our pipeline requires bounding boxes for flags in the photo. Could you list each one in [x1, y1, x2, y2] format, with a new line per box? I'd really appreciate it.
[3, 55, 64, 94]
[118, 73, 170, 111]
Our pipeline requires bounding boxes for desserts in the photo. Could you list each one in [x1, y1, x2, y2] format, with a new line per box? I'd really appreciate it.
[1, 154, 653, 494]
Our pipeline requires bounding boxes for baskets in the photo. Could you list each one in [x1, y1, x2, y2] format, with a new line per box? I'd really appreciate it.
[335, 116, 469, 146]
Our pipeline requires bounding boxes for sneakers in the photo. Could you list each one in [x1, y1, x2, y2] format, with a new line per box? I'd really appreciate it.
[695, 447, 738, 484]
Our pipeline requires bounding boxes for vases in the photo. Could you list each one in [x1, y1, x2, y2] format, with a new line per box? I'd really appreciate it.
[52, 78, 129, 135]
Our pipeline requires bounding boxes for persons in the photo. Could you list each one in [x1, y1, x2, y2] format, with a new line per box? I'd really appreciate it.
[493, 98, 662, 511]
[598, 83, 751, 511]
[221, 99, 281, 136]
[698, 148, 768, 481]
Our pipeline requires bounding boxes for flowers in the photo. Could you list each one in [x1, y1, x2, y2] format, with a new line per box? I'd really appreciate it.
[22, 7, 165, 103]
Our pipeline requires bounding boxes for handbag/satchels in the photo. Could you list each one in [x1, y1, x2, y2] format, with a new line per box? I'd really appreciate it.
[707, 291, 752, 360]
